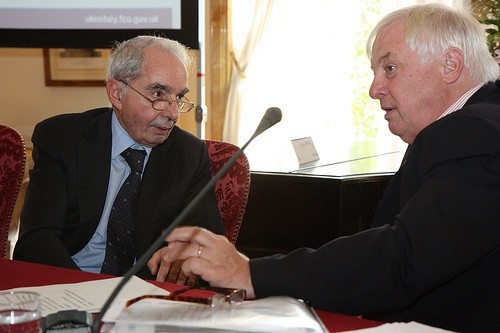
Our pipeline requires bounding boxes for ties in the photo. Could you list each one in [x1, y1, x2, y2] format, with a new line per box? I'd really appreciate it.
[99, 146, 148, 277]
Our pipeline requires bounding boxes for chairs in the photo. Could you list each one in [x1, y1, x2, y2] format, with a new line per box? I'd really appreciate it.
[0, 124, 26, 257]
[201, 138, 253, 243]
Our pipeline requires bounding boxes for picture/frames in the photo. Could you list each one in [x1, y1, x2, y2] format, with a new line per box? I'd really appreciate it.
[43, 47, 114, 87]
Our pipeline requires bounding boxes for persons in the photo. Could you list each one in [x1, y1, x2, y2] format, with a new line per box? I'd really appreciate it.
[13, 35, 226, 287]
[162, 3, 500, 333]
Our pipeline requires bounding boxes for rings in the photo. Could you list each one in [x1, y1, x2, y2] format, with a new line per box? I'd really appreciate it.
[199, 246, 203, 256]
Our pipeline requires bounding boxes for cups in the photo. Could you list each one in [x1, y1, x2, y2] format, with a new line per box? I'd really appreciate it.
[0, 290, 43, 333]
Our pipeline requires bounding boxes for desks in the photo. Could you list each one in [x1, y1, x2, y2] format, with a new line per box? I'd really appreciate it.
[0, 258, 383, 333]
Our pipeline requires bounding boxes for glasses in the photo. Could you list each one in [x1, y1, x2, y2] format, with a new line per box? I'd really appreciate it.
[125, 288, 247, 312]
[116, 79, 195, 113]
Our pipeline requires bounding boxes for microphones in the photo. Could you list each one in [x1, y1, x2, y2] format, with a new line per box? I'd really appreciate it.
[92, 107, 283, 333]
